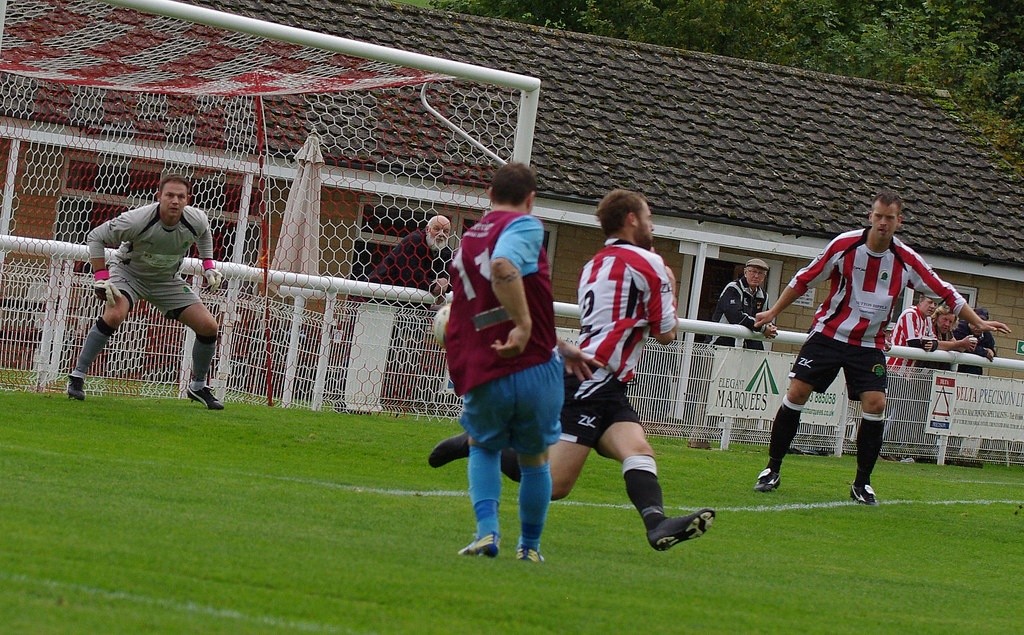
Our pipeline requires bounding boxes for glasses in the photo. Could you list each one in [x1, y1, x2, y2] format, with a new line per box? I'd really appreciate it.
[746, 268, 766, 277]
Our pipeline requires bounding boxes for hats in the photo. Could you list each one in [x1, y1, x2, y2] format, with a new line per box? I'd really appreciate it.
[746, 259, 769, 271]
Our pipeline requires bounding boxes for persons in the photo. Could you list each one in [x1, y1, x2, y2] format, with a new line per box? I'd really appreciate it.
[429, 190, 716, 552]
[67, 174, 224, 411]
[445, 162, 603, 563]
[754, 191, 1011, 506]
[687, 258, 778, 449]
[333, 214, 454, 416]
[879, 294, 996, 467]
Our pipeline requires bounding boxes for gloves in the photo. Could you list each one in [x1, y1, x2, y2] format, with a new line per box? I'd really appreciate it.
[93, 278, 123, 308]
[204, 268, 222, 293]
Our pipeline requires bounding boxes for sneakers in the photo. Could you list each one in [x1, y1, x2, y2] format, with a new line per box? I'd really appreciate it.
[457, 531, 500, 558]
[646, 509, 717, 551]
[66, 372, 85, 399]
[186, 385, 224, 410]
[754, 468, 780, 490]
[850, 480, 876, 504]
[516, 544, 545, 565]
[428, 432, 473, 468]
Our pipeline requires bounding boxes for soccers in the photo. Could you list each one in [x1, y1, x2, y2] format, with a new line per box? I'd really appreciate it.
[433, 302, 451, 347]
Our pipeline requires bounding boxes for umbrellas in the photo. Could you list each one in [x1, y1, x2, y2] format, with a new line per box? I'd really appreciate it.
[267, 128, 326, 294]
